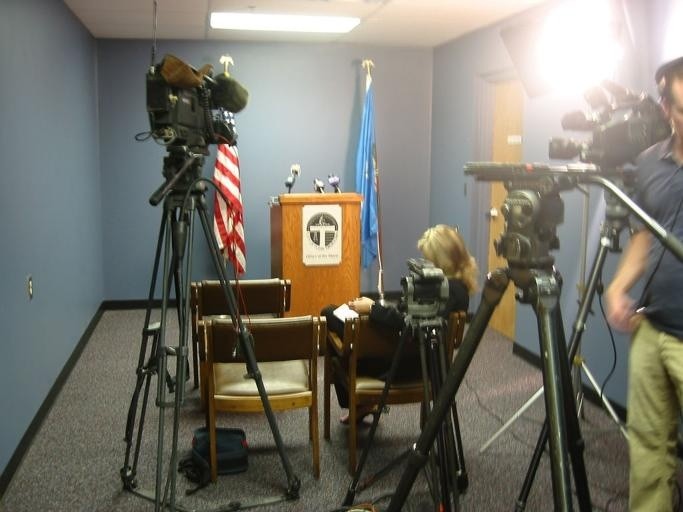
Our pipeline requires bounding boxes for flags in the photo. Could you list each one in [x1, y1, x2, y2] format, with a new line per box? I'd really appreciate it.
[211, 107, 247, 276]
[353, 74, 380, 271]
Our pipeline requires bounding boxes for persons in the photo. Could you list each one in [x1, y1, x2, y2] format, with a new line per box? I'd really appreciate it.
[601, 59, 682, 511]
[318, 223, 484, 425]
[649, 61, 674, 135]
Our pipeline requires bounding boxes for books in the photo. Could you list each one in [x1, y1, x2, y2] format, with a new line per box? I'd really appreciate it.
[329, 301, 360, 329]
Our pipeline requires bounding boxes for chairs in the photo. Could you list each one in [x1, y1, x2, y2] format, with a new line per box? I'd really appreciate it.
[321, 307, 468, 477]
[187, 277, 293, 389]
[196, 312, 331, 485]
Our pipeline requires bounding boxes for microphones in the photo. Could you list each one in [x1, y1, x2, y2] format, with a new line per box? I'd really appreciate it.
[313, 177, 325, 193]
[328, 173, 341, 193]
[284, 164, 301, 193]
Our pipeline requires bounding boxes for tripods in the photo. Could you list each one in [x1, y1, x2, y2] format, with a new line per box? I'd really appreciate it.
[384, 162, 592, 512]
[118, 150, 300, 510]
[341, 316, 470, 511]
[477, 185, 632, 454]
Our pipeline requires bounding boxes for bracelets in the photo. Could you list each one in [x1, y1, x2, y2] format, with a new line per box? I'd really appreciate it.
[366, 300, 376, 316]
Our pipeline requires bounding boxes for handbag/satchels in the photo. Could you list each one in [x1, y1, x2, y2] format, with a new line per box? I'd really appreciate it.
[191, 427, 248, 475]
